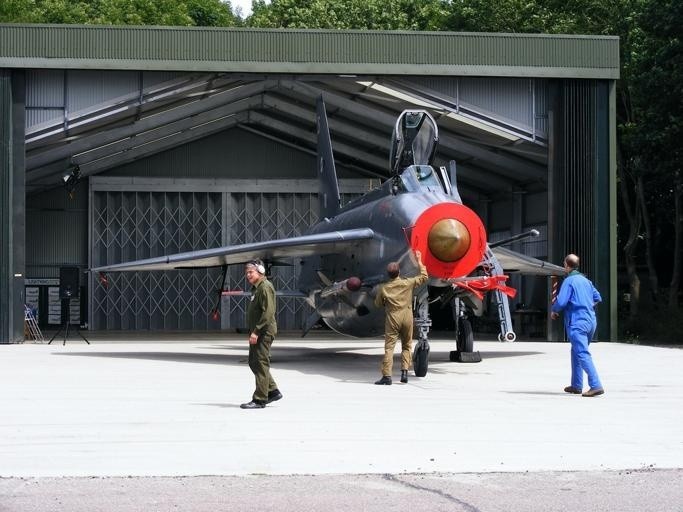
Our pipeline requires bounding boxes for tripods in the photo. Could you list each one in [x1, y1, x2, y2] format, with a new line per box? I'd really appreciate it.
[46, 301, 90, 346]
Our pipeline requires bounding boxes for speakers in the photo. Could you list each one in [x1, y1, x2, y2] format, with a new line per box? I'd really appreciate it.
[59, 267, 79, 299]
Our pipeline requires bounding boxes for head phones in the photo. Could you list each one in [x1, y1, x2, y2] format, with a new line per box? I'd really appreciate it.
[249, 259, 266, 275]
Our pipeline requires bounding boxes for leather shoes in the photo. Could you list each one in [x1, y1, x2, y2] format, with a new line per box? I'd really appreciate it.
[267, 389, 283, 401]
[564, 383, 584, 396]
[373, 376, 393, 385]
[400, 368, 410, 384]
[238, 398, 269, 410]
[580, 387, 607, 399]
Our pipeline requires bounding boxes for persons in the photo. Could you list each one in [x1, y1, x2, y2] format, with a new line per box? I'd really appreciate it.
[238, 257, 283, 408]
[549, 252, 606, 397]
[372, 250, 429, 386]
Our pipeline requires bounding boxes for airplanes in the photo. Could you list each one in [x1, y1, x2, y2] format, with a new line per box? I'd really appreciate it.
[82, 93, 568, 377]
